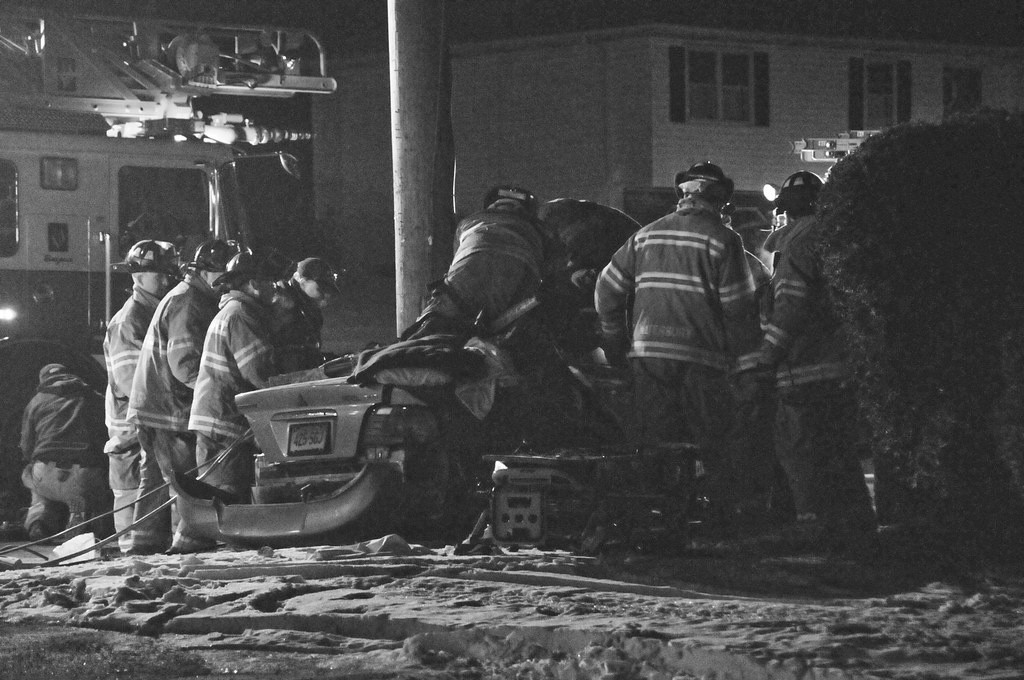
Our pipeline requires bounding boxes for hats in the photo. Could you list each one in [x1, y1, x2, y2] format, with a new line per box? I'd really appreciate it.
[297, 258, 341, 295]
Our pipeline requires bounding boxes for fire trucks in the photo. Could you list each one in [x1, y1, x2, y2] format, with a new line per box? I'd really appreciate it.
[0, 0, 339, 529]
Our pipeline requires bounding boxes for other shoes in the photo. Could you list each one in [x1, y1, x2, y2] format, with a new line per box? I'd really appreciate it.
[28, 519, 53, 546]
[66, 517, 87, 541]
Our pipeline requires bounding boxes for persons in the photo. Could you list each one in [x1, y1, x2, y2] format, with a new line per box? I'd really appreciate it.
[416, 183, 542, 338]
[267, 258, 340, 375]
[102, 240, 182, 553]
[534, 200, 642, 272]
[19, 362, 108, 542]
[167, 250, 279, 555]
[755, 169, 876, 531]
[592, 163, 764, 552]
[128, 239, 251, 556]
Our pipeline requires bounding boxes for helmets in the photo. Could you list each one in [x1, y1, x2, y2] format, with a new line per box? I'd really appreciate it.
[483, 186, 538, 219]
[732, 207, 765, 230]
[775, 171, 822, 217]
[210, 252, 281, 287]
[674, 161, 734, 210]
[36, 363, 77, 390]
[106, 240, 182, 273]
[185, 240, 234, 272]
